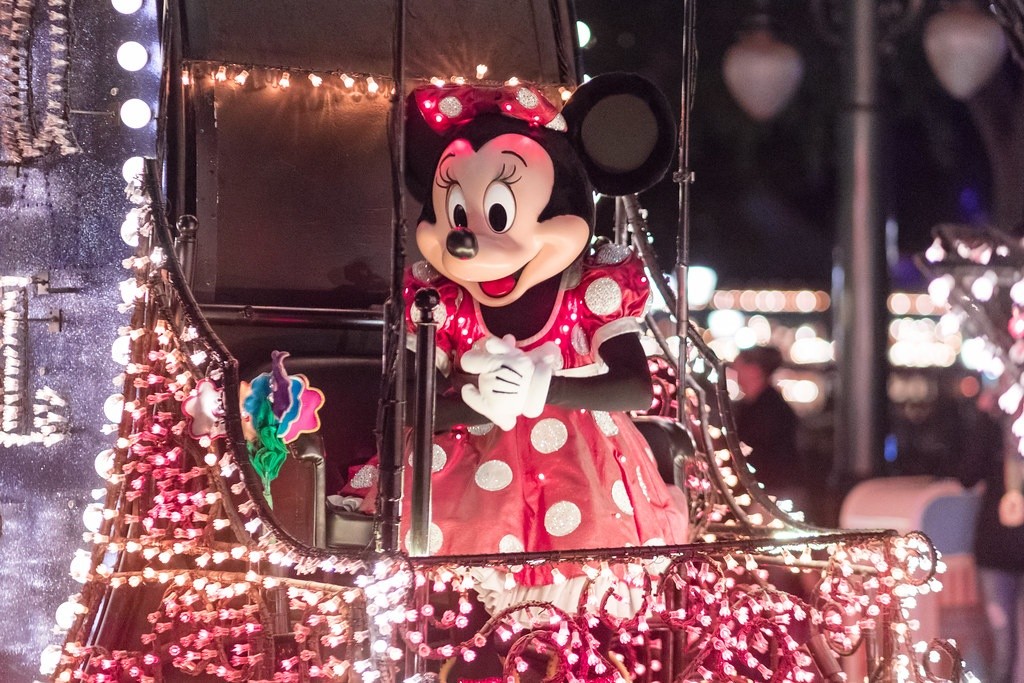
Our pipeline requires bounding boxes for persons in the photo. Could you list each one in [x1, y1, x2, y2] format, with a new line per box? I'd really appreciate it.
[729, 347, 798, 489]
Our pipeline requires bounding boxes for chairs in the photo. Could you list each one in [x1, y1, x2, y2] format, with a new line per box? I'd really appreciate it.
[246, 357, 698, 550]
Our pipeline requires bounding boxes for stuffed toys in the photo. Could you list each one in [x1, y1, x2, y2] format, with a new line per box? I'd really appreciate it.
[326, 73, 688, 561]
